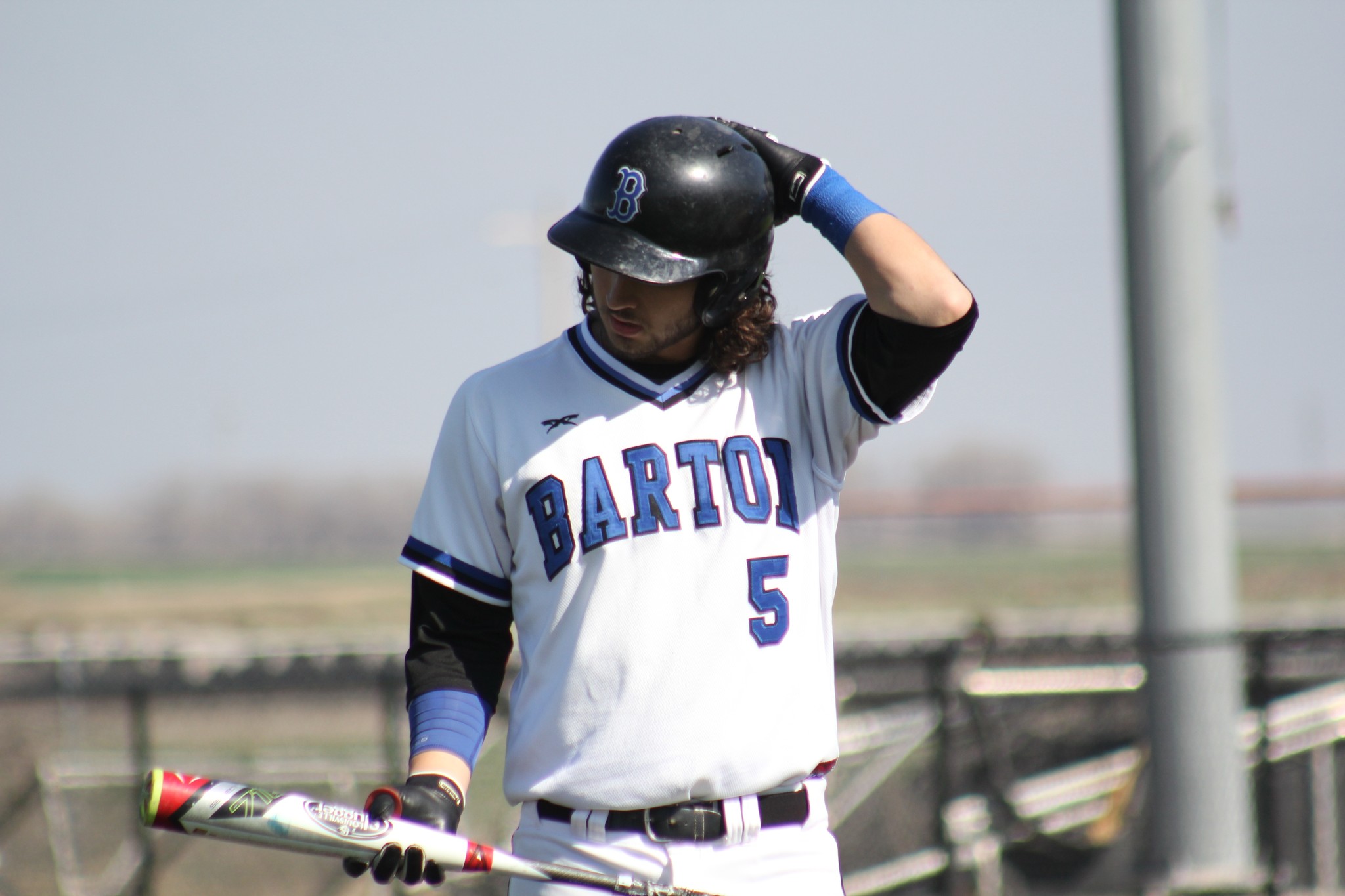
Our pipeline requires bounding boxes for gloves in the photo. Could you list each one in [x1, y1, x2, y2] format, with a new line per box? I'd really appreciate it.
[344, 771, 466, 886]
[705, 117, 826, 227]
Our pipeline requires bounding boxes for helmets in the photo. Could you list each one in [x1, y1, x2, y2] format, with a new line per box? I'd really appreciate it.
[547, 116, 773, 284]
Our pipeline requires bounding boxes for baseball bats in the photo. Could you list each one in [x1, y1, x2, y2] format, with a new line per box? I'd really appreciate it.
[140, 769, 718, 896]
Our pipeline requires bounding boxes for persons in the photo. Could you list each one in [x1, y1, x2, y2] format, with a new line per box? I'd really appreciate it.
[344, 116, 981, 896]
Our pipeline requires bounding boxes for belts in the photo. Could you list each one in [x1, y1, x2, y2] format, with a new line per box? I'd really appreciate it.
[537, 790, 810, 842]
